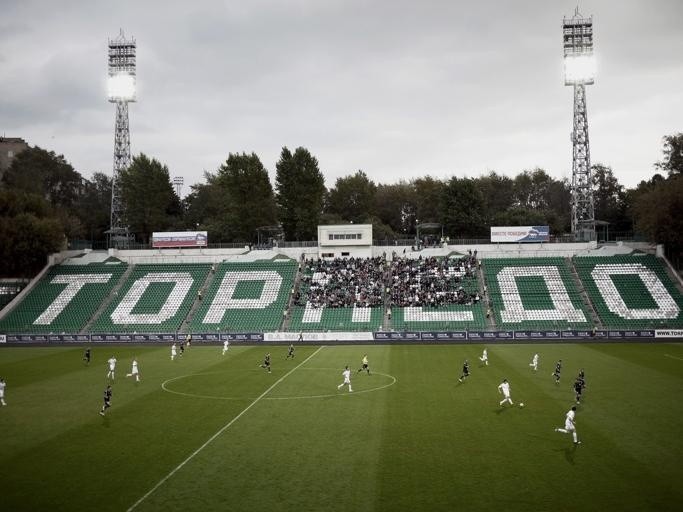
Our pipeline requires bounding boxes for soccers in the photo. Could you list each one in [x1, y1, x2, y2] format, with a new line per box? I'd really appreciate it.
[519, 402, 524, 406]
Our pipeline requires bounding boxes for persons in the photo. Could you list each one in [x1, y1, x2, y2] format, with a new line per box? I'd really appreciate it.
[222, 340, 230, 355]
[478, 347, 488, 365]
[590, 325, 597, 336]
[550, 359, 562, 384]
[294, 249, 488, 309]
[283, 302, 290, 320]
[269, 228, 286, 245]
[106, 355, 117, 379]
[286, 344, 296, 360]
[171, 342, 177, 361]
[528, 351, 540, 371]
[386, 304, 392, 320]
[82, 346, 90, 364]
[179, 342, 185, 358]
[0, 378, 8, 406]
[211, 264, 215, 274]
[578, 368, 586, 388]
[458, 359, 470, 383]
[291, 284, 293, 293]
[414, 233, 448, 248]
[553, 407, 582, 445]
[297, 331, 304, 342]
[337, 365, 354, 392]
[489, 299, 493, 308]
[99, 385, 113, 417]
[355, 353, 373, 376]
[185, 335, 191, 349]
[126, 358, 141, 382]
[487, 307, 491, 318]
[497, 379, 514, 406]
[197, 288, 201, 300]
[257, 352, 272, 374]
[223, 257, 226, 262]
[573, 377, 585, 404]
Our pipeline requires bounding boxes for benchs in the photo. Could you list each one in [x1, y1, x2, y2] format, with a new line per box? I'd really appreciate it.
[0, 254, 683, 333]
